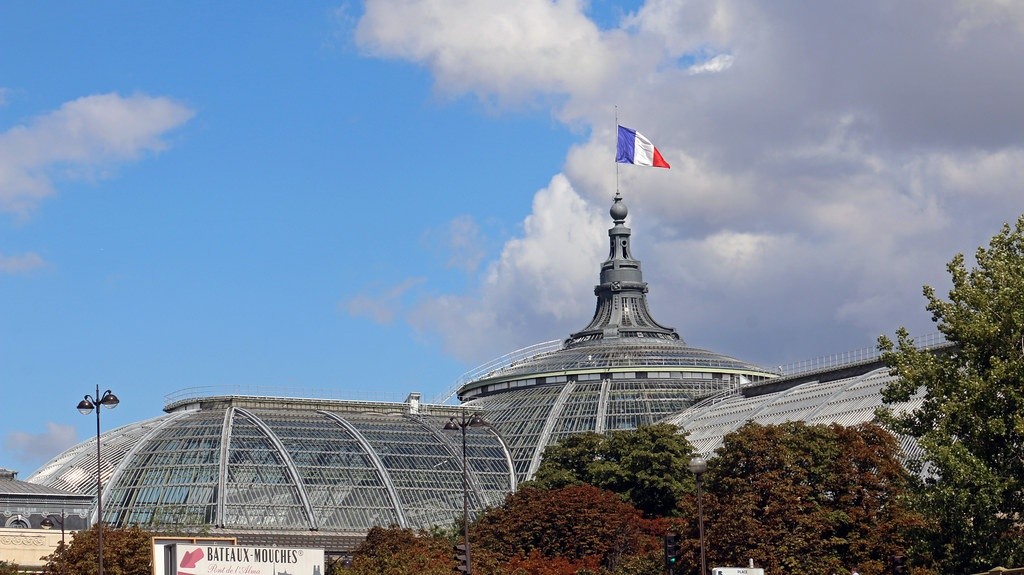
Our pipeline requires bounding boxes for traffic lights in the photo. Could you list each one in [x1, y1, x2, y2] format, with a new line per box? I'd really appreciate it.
[669, 533, 682, 565]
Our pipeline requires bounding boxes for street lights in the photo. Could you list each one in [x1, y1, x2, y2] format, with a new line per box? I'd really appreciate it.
[77, 384, 120, 575]
[41, 509, 64, 562]
[444, 407, 486, 575]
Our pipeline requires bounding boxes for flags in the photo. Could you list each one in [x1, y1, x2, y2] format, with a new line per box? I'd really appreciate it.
[615, 124, 671, 169]
